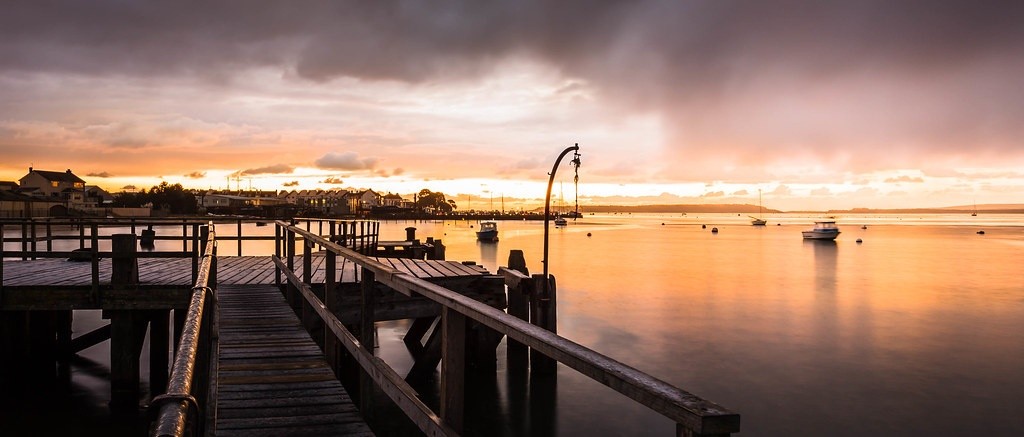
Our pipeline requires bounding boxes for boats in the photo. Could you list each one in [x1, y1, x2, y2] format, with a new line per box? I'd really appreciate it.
[555, 214, 568, 225]
[476, 221, 498, 242]
[801, 221, 842, 240]
[748, 215, 766, 225]
[255, 221, 267, 227]
